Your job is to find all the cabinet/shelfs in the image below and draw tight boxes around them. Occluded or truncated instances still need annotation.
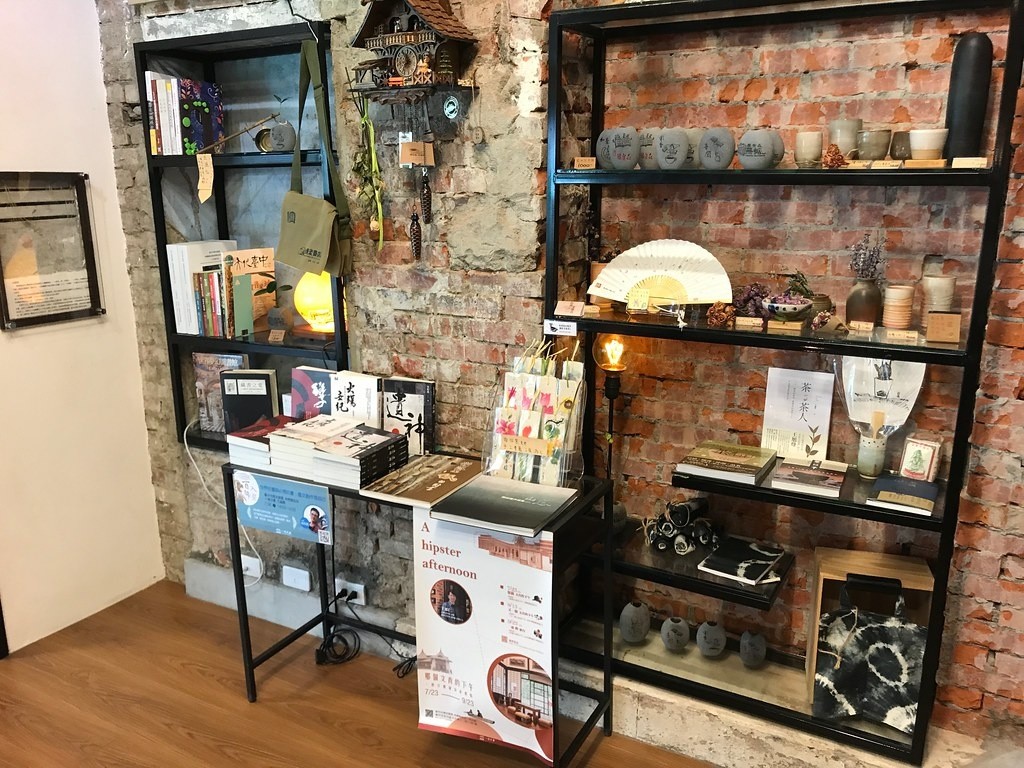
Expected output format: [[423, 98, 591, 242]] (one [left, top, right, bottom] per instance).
[[132, 18, 351, 459], [541, 1, 1023, 766], [220, 449, 615, 767]]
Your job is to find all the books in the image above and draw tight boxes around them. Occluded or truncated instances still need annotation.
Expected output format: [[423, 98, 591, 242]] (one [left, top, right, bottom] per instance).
[[166, 240, 278, 341], [696, 536, 785, 587], [737, 569, 781, 587], [144, 68, 225, 156], [191, 351, 581, 538], [865, 474, 939, 516], [760, 367, 836, 460], [678, 439, 777, 484], [771, 456, 850, 498]]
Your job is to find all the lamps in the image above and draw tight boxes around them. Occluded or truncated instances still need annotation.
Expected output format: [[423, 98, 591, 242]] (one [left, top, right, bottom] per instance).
[[590, 329, 636, 480], [293, 267, 335, 334]]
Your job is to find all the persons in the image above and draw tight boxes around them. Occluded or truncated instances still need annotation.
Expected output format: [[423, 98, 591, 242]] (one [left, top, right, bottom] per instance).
[[440, 589, 466, 624]]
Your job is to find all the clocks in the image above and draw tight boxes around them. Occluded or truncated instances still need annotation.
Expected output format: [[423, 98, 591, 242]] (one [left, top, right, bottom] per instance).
[[394, 45, 418, 78]]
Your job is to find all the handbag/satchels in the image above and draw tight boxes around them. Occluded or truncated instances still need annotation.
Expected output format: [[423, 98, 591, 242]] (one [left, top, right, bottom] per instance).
[[275, 191, 354, 278]]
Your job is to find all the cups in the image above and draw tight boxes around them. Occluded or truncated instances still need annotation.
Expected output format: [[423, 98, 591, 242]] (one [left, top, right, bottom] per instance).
[[910, 127, 950, 159], [920, 275, 958, 332], [856, 129, 892, 159], [795, 132, 823, 168], [882, 283, 915, 329], [858, 434, 888, 479], [828, 118, 863, 160]]
